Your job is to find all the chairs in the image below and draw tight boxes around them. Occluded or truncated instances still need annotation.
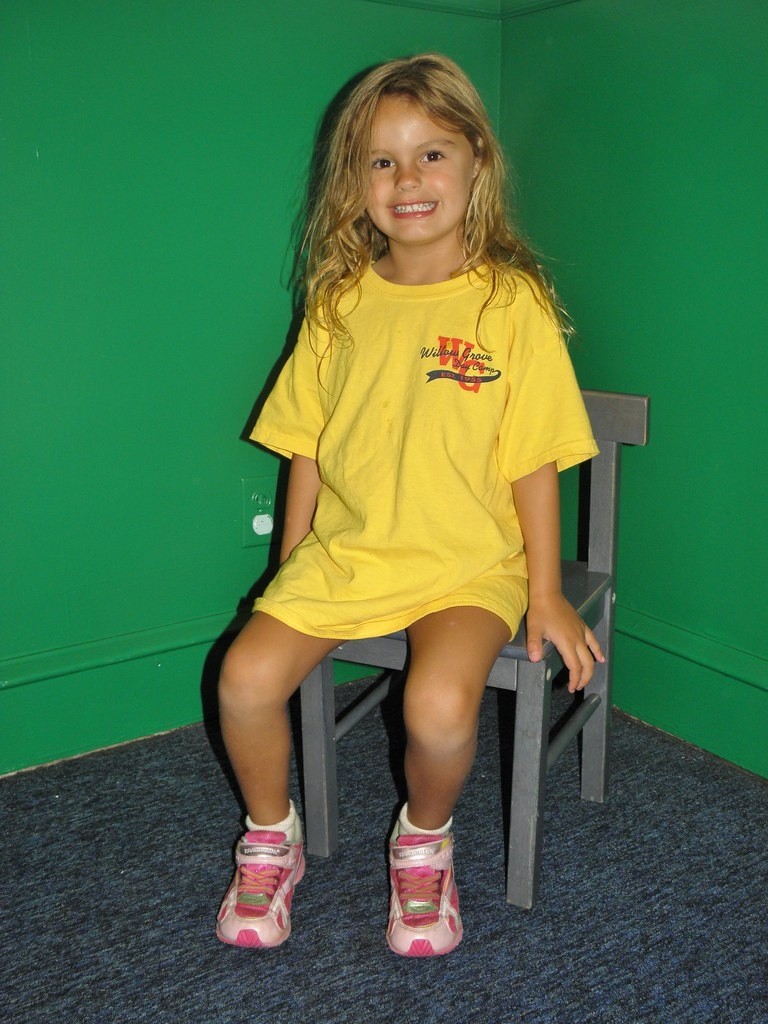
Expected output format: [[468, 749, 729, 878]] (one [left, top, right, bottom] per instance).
[[301, 389, 651, 910]]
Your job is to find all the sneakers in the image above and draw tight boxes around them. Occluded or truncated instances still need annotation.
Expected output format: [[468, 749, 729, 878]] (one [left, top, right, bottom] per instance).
[[385, 818, 463, 957], [215, 812, 306, 949]]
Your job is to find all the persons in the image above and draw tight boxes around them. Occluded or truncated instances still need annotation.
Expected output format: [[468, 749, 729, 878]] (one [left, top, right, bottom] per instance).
[[216, 53, 608, 958]]
